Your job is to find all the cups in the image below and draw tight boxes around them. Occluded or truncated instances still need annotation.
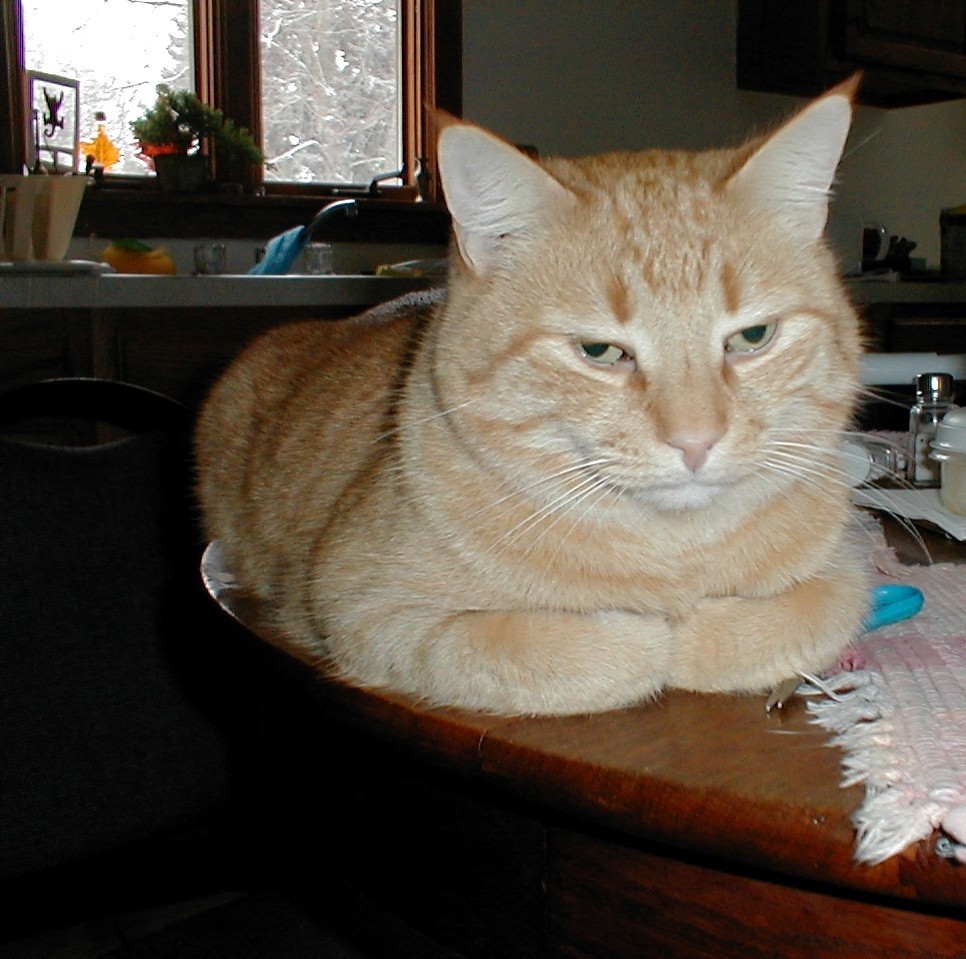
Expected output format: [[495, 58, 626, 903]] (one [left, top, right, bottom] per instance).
[[827, 200, 889, 276]]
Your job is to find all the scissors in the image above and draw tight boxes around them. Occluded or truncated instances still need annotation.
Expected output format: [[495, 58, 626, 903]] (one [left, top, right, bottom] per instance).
[[764, 582, 924, 710]]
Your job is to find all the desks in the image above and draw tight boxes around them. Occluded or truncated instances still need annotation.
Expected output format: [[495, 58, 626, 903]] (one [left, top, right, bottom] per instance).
[[201, 433, 965, 959]]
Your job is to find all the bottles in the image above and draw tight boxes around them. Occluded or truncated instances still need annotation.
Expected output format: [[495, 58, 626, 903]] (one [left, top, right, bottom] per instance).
[[843, 440, 904, 487], [907, 372, 959, 488]]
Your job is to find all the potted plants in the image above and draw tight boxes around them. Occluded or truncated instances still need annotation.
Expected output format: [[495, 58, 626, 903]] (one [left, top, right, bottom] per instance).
[[126, 87, 263, 192]]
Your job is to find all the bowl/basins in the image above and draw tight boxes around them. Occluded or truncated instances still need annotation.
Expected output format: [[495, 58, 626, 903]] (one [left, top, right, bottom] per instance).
[[928, 407, 966, 516]]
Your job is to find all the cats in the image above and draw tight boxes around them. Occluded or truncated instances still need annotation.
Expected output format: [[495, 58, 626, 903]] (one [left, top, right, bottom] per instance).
[[193, 70, 946, 719]]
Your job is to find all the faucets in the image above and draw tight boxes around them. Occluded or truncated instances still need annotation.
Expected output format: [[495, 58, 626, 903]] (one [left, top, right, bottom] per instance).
[[255, 197, 358, 273]]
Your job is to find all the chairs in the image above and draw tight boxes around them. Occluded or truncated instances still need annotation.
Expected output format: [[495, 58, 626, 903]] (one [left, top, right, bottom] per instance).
[[0, 377, 392, 959]]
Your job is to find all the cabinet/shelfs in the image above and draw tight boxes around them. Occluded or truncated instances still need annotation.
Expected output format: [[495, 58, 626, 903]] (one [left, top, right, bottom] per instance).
[[735, 0, 966, 109]]
